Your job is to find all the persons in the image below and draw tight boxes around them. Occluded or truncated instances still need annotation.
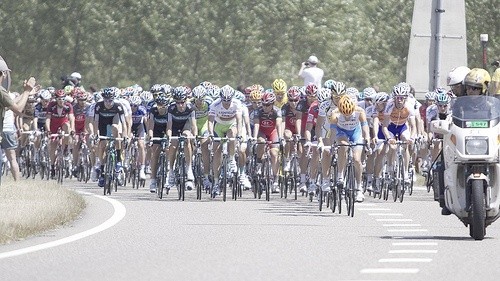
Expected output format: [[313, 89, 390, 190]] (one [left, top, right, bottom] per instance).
[[298, 55, 325, 85], [0, 56, 500, 201]]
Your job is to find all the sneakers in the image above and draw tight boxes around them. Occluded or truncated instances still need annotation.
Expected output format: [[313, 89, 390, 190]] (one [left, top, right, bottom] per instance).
[[0, 154, 453, 215]]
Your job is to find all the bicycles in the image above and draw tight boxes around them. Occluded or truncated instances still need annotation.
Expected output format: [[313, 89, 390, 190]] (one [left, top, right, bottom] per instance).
[[2, 126, 431, 218]]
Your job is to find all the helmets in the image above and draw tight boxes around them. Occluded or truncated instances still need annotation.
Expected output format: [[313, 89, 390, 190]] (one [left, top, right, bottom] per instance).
[[0, 55, 500, 117]]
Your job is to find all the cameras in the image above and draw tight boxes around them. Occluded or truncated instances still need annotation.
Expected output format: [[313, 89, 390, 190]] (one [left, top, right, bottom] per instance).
[[62, 76, 77, 86], [305, 62, 309, 65]]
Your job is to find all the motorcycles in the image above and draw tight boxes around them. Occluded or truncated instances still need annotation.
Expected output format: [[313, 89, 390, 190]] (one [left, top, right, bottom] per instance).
[[426, 95, 500, 240]]
